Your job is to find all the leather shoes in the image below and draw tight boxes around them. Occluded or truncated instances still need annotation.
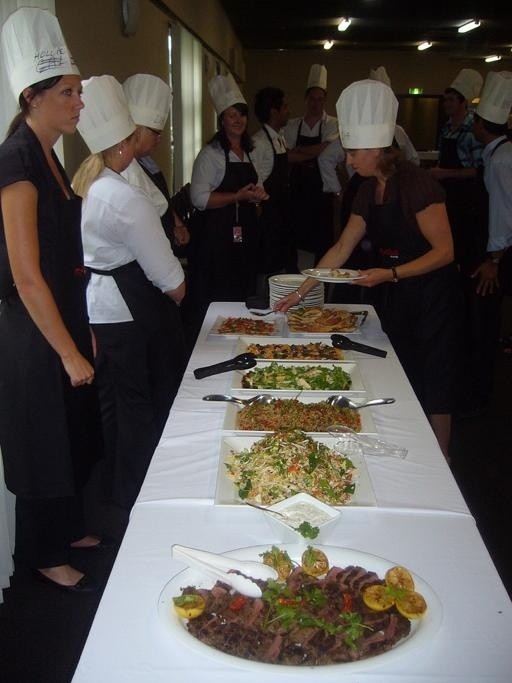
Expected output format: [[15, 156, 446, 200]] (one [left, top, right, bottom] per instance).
[[63, 536, 113, 553], [23, 568, 99, 597]]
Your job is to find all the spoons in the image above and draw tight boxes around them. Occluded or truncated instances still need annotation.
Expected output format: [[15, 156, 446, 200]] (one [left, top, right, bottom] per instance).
[[202, 394, 279, 407], [249, 311, 276, 316], [326, 395, 395, 410]]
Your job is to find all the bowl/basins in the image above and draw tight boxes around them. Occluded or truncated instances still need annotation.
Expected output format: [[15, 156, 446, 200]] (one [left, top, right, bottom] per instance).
[[263, 493, 342, 543]]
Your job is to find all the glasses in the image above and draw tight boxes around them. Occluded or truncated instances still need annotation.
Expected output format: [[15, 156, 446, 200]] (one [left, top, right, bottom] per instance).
[[145, 127, 161, 137]]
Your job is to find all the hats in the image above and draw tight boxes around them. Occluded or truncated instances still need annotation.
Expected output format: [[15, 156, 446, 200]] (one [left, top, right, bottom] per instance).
[[307, 63, 327, 90], [123, 72, 173, 130], [1, 6, 80, 108], [366, 65, 392, 89], [207, 71, 247, 119], [76, 74, 137, 154], [335, 78, 399, 149], [450, 68, 482, 102], [475, 70, 512, 124]]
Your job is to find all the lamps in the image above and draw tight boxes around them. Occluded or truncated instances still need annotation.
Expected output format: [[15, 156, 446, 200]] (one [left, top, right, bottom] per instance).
[[484, 54, 502, 64], [457, 18, 482, 34], [417, 40, 432, 51], [323, 39, 336, 50], [337, 14, 351, 32]]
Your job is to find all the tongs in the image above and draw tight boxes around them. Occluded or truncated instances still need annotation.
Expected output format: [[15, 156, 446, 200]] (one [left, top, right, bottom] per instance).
[[194, 352, 256, 380], [329, 333, 389, 359], [168, 542, 279, 601]]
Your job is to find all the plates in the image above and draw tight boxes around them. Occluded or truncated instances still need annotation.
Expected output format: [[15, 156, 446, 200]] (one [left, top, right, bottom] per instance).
[[232, 337, 356, 363], [269, 274, 326, 310], [222, 394, 379, 437], [230, 360, 367, 394], [212, 434, 382, 509], [208, 313, 286, 336], [301, 269, 368, 284], [157, 542, 442, 679], [285, 309, 362, 334]]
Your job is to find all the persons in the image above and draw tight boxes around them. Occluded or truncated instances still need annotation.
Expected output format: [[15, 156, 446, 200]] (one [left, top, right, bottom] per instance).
[[0, 5, 190, 595], [192, 65, 510, 466]]
